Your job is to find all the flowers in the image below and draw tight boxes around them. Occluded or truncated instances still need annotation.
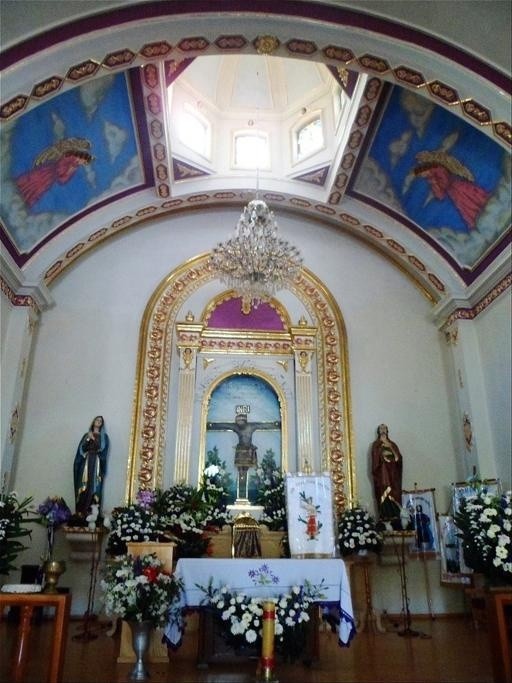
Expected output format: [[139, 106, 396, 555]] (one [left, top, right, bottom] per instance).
[[340, 504, 385, 555], [35, 494, 72, 589], [197, 579, 324, 652], [103, 553, 183, 625], [106, 465, 228, 560], [457, 486, 511, 584], [255, 467, 293, 557]]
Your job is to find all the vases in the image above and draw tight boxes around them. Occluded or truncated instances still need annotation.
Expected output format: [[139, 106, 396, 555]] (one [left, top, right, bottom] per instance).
[[127, 621, 157, 682], [40, 557, 67, 595], [125, 541, 178, 577]]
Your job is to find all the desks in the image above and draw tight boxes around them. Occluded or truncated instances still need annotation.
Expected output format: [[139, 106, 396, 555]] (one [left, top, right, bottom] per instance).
[[176, 556, 346, 670], [0, 588, 73, 683]]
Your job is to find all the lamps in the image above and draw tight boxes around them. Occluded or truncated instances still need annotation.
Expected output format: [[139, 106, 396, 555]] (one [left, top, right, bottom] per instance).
[[207, 168, 306, 310]]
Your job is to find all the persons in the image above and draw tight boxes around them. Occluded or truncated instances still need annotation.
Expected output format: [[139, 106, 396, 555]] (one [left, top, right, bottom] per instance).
[[205, 414, 280, 496], [73, 416, 110, 525], [370, 424, 403, 531], [407, 505, 434, 549]]
